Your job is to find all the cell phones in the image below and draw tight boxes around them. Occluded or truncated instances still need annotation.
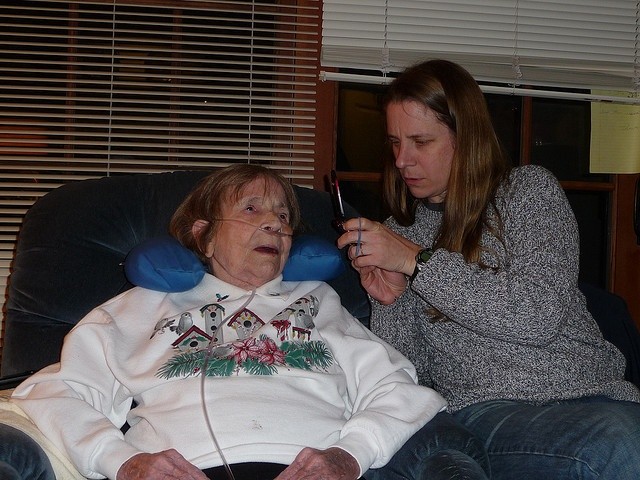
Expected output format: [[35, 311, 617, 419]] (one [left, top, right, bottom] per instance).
[[331, 170, 358, 250]]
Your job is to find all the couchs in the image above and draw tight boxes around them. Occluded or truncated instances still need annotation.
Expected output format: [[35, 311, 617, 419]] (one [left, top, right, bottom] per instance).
[[1, 167, 640, 480]]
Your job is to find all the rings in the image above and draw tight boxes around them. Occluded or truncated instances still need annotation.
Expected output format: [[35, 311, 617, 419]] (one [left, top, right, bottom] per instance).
[[360, 243, 365, 257]]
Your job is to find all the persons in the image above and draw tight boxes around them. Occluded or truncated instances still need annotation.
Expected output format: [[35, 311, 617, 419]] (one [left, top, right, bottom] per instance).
[[10, 163, 448, 479], [338, 60, 640, 480]]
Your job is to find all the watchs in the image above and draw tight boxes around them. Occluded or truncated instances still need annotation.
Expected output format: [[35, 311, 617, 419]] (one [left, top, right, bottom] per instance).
[[410, 246, 434, 283]]
